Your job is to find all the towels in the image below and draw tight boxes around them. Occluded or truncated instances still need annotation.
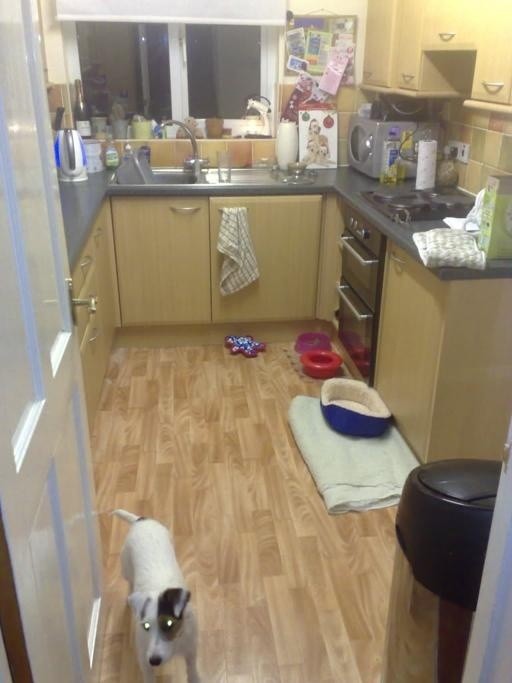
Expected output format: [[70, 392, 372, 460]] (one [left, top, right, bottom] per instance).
[[382, 460, 504, 683]]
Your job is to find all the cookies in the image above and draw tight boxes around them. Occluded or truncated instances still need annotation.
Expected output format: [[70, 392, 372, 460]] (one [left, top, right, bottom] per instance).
[[346, 114, 441, 180]]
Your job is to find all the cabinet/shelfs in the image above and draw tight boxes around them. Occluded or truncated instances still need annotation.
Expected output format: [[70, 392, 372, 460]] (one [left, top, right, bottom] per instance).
[[106, 140, 151, 169], [463, 0, 512, 108], [72, 79, 92, 139], [357, 3, 467, 101], [380, 128, 400, 187], [373, 232, 512, 468], [106, 166, 334, 345], [421, 1, 479, 50], [57, 183, 116, 417]]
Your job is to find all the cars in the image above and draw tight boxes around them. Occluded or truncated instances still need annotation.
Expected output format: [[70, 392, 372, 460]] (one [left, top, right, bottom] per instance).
[[91, 115, 164, 140], [274, 122, 299, 172], [205, 117, 224, 139], [217, 150, 233, 181], [288, 162, 307, 182]]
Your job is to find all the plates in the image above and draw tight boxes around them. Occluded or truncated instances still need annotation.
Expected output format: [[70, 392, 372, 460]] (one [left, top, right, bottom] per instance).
[[373, 232, 512, 468], [463, 0, 512, 108], [357, 3, 467, 101], [421, 1, 479, 50]]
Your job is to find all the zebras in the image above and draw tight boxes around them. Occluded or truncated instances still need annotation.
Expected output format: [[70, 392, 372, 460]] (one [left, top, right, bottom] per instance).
[[335, 231, 384, 387]]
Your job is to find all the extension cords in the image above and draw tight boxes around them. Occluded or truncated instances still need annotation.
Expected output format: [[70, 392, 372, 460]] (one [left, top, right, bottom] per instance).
[[54, 129, 89, 183]]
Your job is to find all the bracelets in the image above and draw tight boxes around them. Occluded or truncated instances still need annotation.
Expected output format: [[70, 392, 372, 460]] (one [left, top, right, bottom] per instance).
[[339, 329, 371, 377], [296, 331, 343, 379]]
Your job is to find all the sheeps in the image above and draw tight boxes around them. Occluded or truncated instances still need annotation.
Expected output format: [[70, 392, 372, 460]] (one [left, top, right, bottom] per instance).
[[153, 119, 199, 159]]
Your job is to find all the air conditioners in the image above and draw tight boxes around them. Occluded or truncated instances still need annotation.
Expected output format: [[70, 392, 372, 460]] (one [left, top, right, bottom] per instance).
[[108, 509, 200, 683]]
